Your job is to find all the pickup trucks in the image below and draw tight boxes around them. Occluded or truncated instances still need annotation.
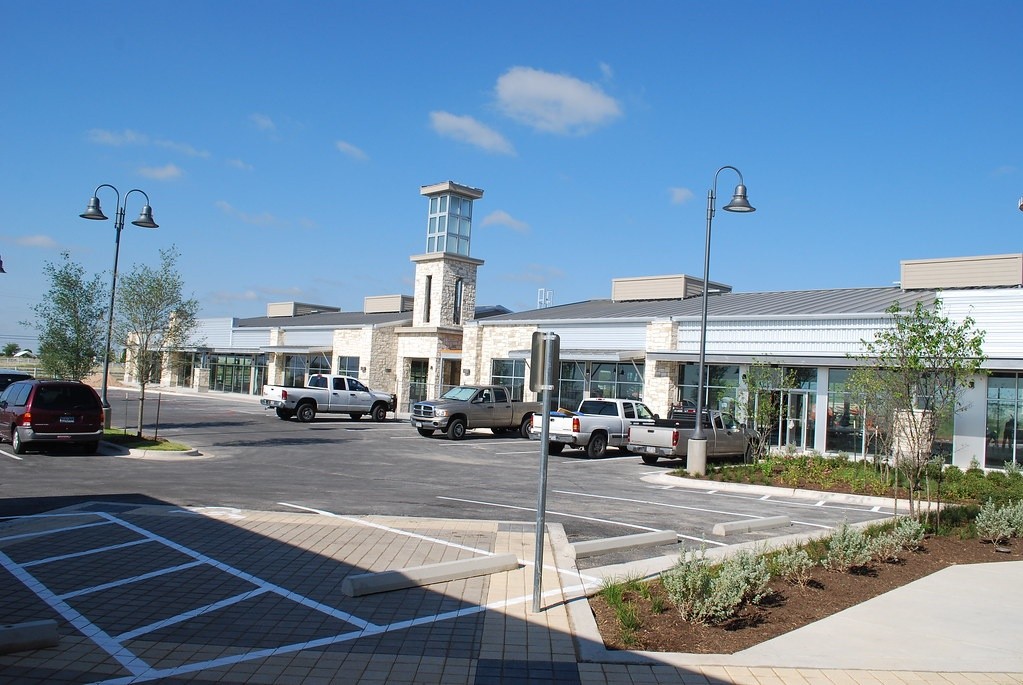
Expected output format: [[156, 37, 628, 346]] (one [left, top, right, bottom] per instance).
[[527, 396, 662, 459], [625, 402, 761, 467], [408, 384, 545, 441], [259, 373, 399, 424]]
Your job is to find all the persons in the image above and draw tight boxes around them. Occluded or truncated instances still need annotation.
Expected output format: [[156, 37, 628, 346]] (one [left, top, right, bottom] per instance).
[[1002, 418, 1014, 448]]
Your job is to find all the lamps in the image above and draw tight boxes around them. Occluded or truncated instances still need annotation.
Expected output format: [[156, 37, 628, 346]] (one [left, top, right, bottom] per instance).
[[361, 367, 366, 370], [463, 369, 470, 373]]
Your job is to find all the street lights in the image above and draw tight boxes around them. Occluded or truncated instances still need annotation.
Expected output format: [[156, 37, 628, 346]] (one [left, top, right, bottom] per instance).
[[77, 183, 159, 408], [691, 165, 757, 440]]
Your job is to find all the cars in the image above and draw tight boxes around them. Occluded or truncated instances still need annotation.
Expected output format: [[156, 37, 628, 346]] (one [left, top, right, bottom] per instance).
[[0, 367, 34, 397]]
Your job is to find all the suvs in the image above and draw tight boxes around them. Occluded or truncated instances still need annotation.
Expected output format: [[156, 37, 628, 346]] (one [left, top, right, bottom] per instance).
[[0, 377, 105, 454]]
